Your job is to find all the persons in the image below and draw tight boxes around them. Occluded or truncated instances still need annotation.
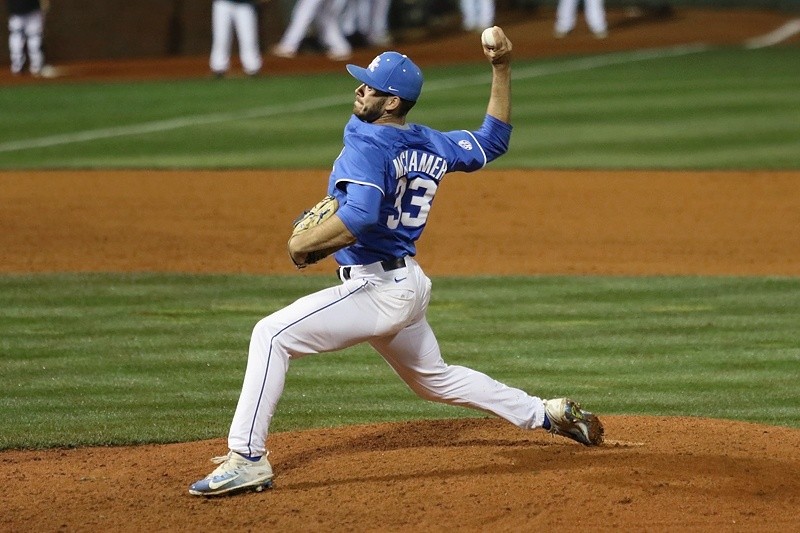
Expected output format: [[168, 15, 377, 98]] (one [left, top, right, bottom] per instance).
[[6, 0, 49, 75], [210, 0, 395, 78], [552, 0, 609, 41], [189, 26, 605, 497], [459, 0, 496, 34]]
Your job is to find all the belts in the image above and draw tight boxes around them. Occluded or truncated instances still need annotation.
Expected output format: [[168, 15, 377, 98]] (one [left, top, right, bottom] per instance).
[[337, 258, 407, 279]]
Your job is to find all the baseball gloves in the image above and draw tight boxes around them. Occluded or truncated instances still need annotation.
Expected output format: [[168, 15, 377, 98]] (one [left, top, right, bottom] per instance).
[[288, 194, 355, 266]]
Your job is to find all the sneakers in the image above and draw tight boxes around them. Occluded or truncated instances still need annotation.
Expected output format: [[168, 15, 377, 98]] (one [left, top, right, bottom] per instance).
[[543, 398, 605, 448], [189, 452, 275, 499]]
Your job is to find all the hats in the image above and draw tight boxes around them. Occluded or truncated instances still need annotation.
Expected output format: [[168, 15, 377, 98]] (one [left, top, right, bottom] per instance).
[[346, 51, 423, 102]]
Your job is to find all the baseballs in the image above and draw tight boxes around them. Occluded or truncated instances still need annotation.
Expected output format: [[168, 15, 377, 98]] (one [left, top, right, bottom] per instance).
[[481, 27, 501, 50]]
